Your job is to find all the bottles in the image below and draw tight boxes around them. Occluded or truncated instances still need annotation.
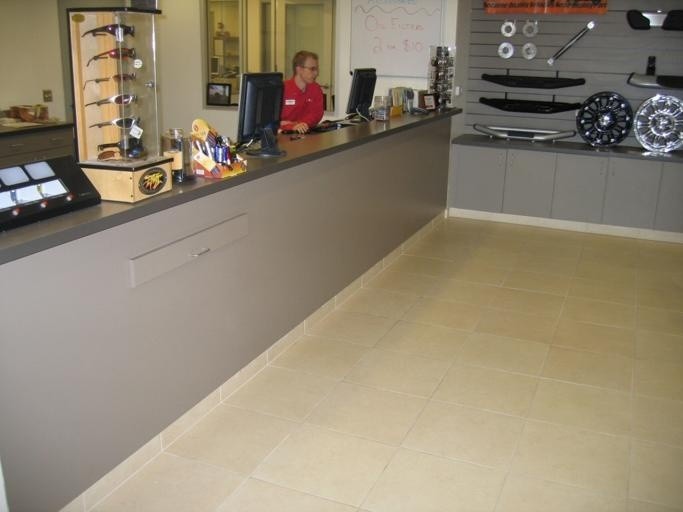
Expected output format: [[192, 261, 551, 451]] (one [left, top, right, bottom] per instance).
[[374, 96, 390, 121], [166, 127, 185, 184]]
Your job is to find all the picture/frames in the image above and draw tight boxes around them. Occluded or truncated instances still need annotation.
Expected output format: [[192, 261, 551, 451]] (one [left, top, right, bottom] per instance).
[[207, 83, 232, 106]]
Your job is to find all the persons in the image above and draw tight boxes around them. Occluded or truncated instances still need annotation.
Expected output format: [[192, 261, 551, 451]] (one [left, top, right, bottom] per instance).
[[277, 48, 327, 136]]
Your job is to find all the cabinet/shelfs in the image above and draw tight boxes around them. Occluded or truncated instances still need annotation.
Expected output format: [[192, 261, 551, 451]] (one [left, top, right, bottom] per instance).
[[212, 37, 239, 75], [0, 123, 74, 169]]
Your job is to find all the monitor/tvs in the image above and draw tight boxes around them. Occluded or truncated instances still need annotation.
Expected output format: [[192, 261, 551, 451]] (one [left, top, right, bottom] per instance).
[[236, 72, 287, 158], [346, 68, 377, 123], [211, 56, 220, 75]]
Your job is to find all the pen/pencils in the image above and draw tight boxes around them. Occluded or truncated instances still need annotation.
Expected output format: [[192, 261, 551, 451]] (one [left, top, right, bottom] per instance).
[[195, 140, 231, 167]]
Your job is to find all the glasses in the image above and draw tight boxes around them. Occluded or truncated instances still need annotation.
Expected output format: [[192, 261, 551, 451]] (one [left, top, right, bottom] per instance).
[[86, 48, 137, 67], [298, 65, 319, 71], [98, 138, 145, 160], [82, 24, 135, 38], [89, 117, 144, 130], [85, 94, 137, 107], [83, 71, 136, 90]]
